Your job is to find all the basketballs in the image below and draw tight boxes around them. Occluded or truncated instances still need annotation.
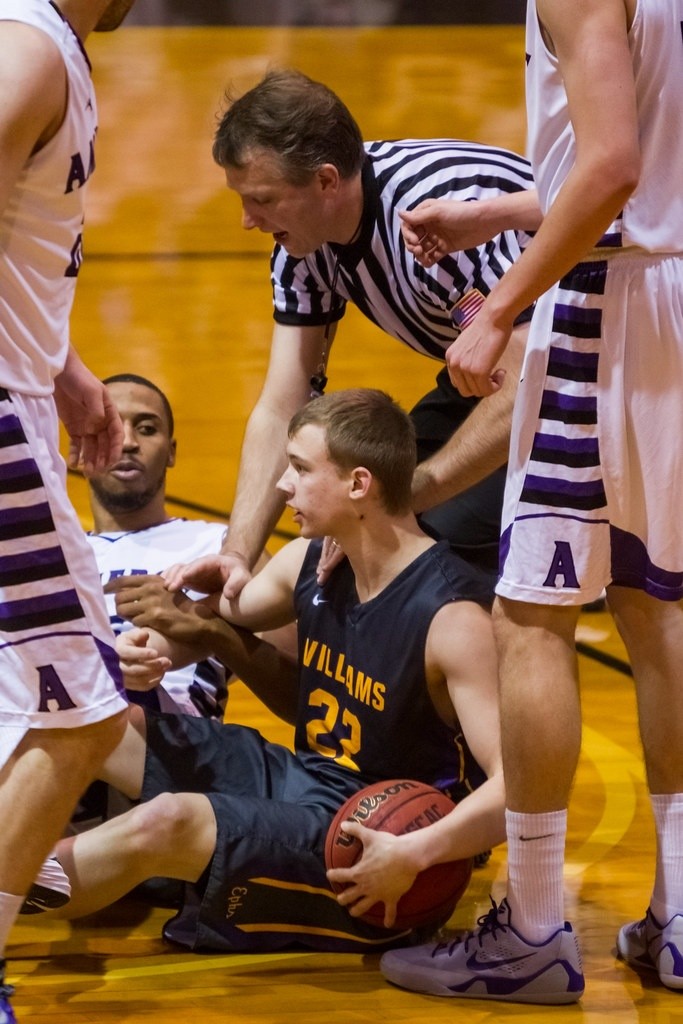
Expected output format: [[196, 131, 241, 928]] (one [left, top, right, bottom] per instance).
[[325, 777, 473, 932]]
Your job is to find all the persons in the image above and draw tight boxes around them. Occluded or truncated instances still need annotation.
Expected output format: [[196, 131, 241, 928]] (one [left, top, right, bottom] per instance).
[[0, 0, 683, 1024]]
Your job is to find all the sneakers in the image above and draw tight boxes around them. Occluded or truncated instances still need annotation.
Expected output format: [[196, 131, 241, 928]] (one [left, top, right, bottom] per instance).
[[380, 894, 585, 1005], [19, 852, 71, 915], [0, 959, 17, 1024], [616, 907, 683, 989]]
